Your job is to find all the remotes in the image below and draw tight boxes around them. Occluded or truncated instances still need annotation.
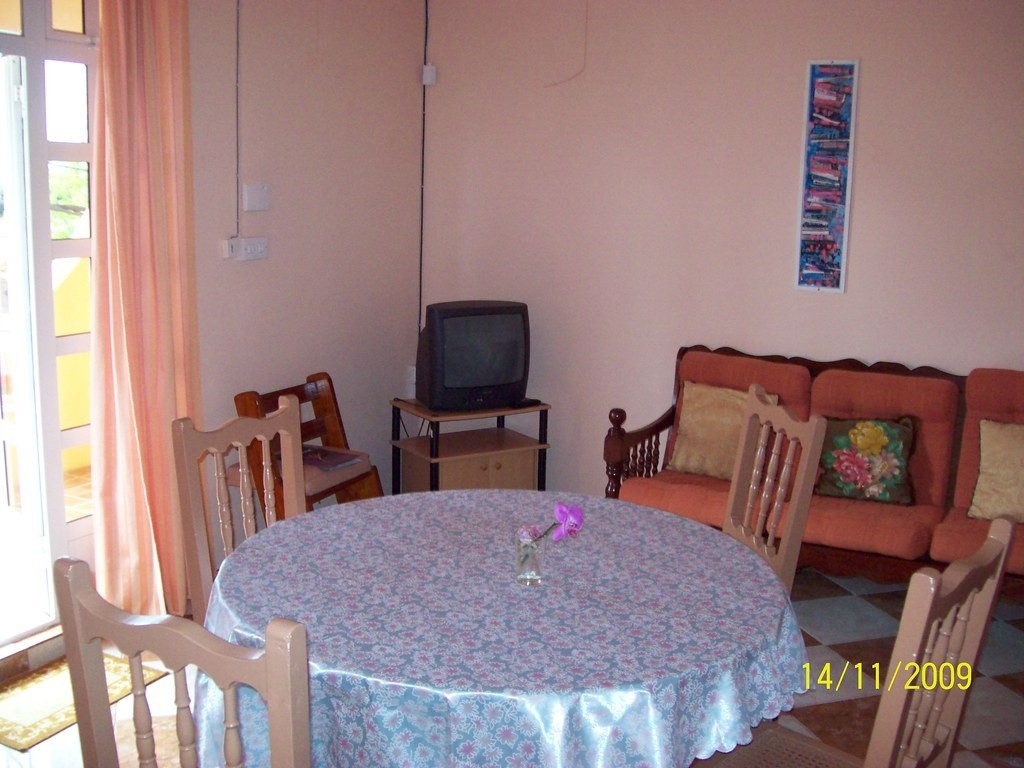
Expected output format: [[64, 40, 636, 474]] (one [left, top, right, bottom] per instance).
[[512, 398, 542, 410]]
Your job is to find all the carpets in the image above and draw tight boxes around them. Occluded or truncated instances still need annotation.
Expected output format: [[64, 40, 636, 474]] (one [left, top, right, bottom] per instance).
[[0, 652, 169, 752]]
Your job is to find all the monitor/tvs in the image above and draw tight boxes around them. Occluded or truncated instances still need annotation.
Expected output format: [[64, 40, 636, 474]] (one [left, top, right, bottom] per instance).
[[416, 300, 529, 411]]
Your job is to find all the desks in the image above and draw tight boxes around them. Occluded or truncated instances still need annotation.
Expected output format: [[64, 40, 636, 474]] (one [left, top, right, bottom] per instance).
[[193, 489, 816, 768]]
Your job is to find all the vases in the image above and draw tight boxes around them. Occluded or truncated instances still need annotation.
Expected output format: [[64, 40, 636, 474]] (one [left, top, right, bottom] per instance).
[[517, 538, 545, 586]]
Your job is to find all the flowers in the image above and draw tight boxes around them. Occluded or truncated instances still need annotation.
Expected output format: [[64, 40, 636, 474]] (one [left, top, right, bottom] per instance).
[[518, 504, 584, 566]]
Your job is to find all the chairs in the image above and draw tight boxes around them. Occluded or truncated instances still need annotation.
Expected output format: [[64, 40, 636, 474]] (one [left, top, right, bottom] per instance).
[[172, 394, 306, 626], [689, 515, 1018, 768], [723, 383, 828, 594], [54, 557, 310, 768], [227, 372, 384, 529]]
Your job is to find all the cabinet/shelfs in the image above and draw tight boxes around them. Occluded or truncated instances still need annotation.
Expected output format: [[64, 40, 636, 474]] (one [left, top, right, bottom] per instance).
[[389, 398, 552, 495]]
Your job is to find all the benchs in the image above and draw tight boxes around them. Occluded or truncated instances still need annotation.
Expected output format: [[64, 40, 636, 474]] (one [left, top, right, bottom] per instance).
[[604, 345, 1023, 601]]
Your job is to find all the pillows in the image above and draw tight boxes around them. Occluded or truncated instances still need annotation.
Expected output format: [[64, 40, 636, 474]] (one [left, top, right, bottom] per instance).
[[665, 381, 778, 481], [814, 417, 914, 505], [967, 419, 1024, 524]]
